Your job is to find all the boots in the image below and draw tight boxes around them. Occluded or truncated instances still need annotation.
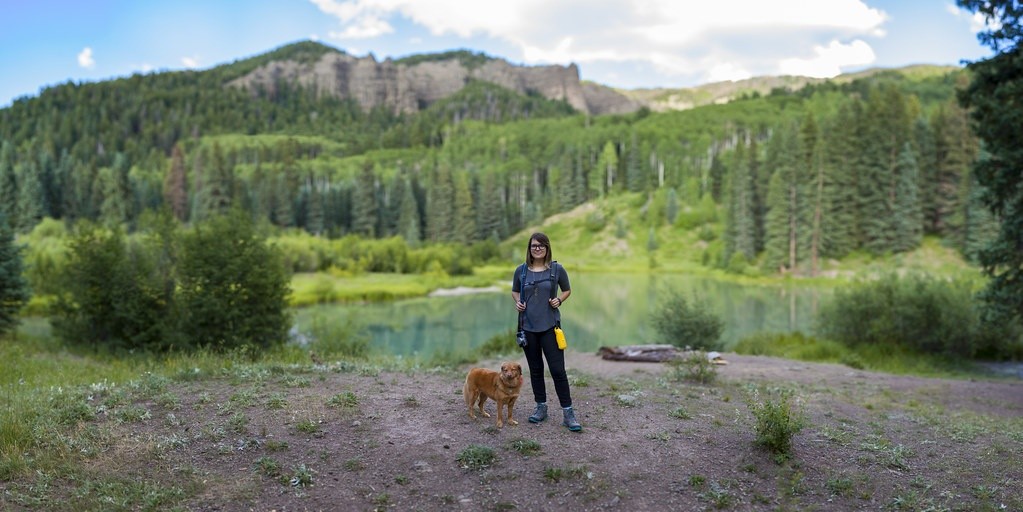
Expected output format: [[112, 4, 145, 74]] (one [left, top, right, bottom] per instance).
[[528, 403, 548, 423], [562, 407, 581, 431]]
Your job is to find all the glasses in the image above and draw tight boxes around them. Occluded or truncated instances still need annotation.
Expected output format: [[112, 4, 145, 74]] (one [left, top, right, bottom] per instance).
[[530, 244, 547, 250]]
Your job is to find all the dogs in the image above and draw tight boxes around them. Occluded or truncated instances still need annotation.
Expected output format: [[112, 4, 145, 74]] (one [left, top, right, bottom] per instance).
[[462, 361, 523, 428]]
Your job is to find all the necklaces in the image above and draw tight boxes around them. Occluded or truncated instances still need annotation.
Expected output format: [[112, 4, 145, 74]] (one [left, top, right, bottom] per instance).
[[532, 264, 545, 296]]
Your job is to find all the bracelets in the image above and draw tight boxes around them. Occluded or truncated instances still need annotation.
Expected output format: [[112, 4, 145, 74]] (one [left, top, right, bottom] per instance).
[[556, 297, 562, 307]]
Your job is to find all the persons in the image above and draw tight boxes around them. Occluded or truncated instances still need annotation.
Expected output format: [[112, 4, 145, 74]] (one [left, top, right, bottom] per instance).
[[512, 232, 583, 431]]
